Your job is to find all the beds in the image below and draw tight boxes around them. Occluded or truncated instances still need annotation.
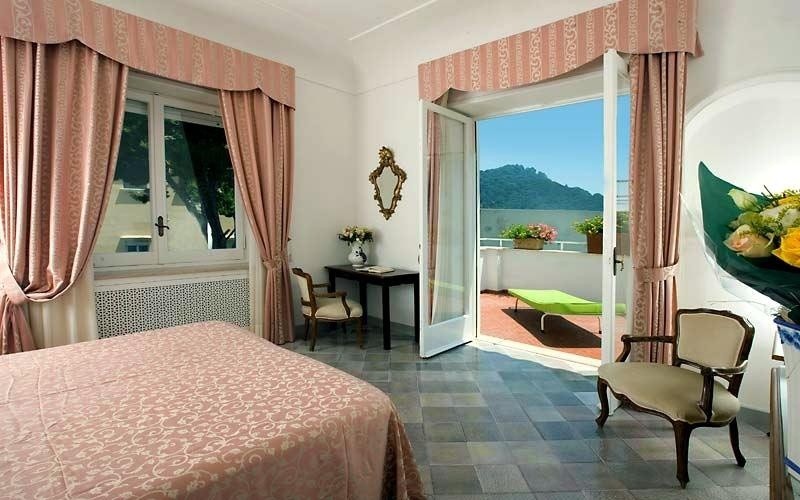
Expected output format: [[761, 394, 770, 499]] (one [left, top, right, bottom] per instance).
[[0, 318, 430, 500]]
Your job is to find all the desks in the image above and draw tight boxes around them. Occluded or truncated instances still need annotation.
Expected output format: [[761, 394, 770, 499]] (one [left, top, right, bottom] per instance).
[[323, 263, 420, 350]]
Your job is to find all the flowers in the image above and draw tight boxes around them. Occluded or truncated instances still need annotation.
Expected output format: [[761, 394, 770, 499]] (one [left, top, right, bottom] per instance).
[[335, 223, 375, 248], [497, 221, 560, 244], [724, 182, 800, 273], [571, 210, 629, 237]]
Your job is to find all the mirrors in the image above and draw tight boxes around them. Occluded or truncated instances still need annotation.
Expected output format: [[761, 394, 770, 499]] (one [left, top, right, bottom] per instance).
[[368, 145, 408, 222]]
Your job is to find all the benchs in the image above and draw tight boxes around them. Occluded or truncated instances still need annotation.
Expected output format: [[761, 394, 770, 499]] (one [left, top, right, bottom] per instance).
[[507, 288, 627, 334]]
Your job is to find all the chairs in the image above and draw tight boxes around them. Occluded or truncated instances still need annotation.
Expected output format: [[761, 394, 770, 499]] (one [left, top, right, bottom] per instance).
[[291, 267, 363, 352], [595, 305, 756, 489]]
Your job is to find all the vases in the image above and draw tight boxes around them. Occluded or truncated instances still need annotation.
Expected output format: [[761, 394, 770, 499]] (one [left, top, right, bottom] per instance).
[[347, 240, 368, 269], [587, 233, 630, 256], [770, 312, 800, 483], [513, 235, 544, 250]]
